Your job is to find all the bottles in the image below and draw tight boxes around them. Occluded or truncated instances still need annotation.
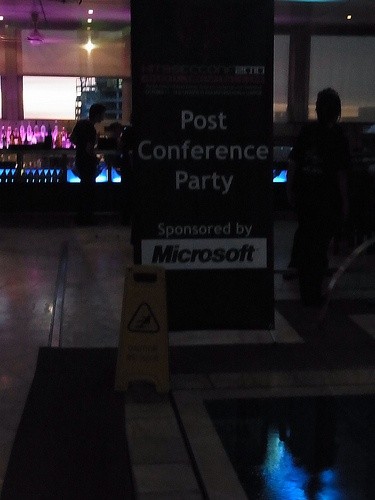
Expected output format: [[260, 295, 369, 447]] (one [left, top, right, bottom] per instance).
[[0, 169, 58, 184], [0, 120, 68, 150]]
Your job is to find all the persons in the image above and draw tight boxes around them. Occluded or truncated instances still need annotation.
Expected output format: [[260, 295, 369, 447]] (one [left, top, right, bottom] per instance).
[[274, 85, 364, 315], [68, 103, 109, 229], [110, 112, 135, 224]]
